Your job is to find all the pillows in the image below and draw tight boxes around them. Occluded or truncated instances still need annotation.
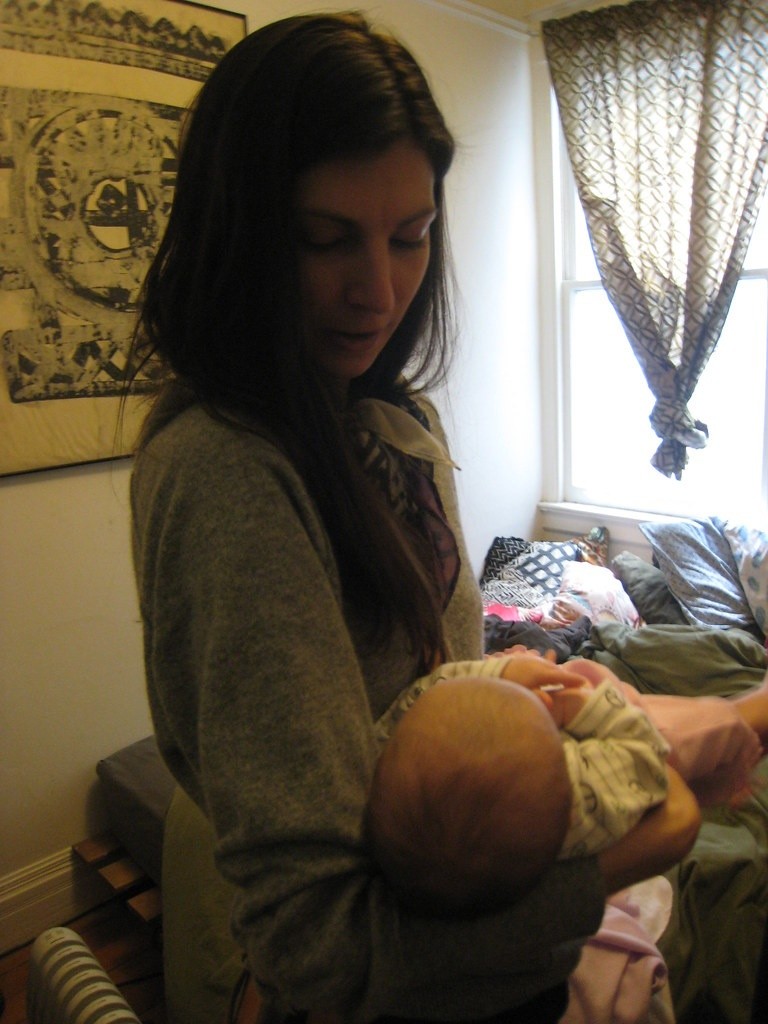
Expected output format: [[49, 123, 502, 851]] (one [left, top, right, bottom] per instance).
[[479, 523, 610, 614]]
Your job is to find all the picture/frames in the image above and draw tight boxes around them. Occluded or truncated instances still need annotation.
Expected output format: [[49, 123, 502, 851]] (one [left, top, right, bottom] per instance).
[[0, 1, 248, 480]]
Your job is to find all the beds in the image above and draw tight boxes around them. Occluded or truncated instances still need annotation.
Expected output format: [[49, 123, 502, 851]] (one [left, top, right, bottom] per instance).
[[68, 509, 768, 1024]]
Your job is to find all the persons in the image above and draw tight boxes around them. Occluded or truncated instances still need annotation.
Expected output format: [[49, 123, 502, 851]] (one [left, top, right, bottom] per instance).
[[107, 9, 768, 1024]]
